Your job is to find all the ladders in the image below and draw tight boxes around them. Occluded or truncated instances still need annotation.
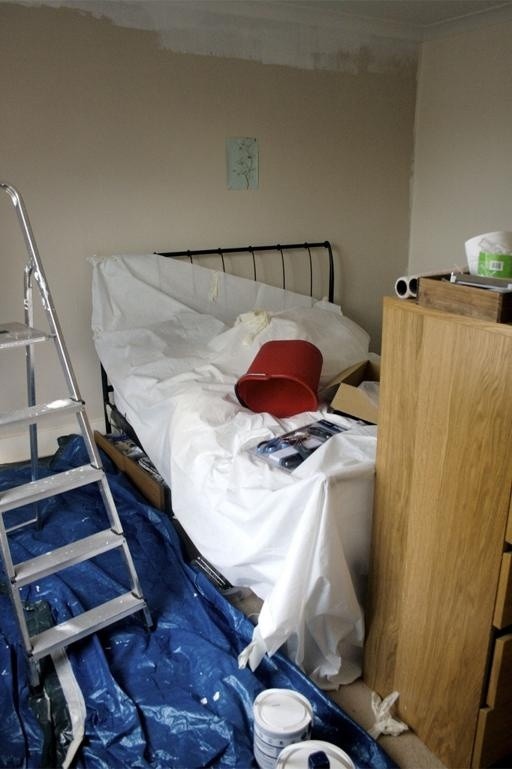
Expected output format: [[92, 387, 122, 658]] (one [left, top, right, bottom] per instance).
[[0, 183, 154, 698]]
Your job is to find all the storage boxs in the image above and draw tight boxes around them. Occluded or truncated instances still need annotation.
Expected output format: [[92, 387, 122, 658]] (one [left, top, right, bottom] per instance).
[[415, 271, 512, 324], [317, 357, 380, 426]]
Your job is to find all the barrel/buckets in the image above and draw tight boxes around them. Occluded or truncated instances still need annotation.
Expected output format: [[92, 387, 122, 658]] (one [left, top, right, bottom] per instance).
[[236, 340, 325, 418]]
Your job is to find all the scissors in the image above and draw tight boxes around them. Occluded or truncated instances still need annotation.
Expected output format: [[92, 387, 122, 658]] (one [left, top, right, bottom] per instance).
[[256, 437, 307, 453]]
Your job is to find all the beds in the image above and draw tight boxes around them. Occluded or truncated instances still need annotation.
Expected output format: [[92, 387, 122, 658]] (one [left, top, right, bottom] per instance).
[[89, 240, 379, 691]]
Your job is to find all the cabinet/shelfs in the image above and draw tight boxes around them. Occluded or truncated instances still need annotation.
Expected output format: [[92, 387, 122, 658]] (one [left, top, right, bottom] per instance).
[[358, 293, 512, 769]]
